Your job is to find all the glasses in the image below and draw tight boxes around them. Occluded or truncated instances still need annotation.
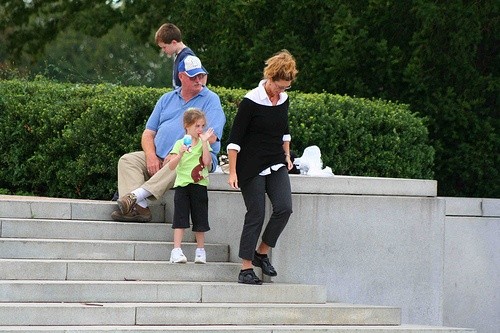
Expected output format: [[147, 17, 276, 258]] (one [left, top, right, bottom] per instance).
[[273, 80, 291, 89], [190, 74, 204, 81]]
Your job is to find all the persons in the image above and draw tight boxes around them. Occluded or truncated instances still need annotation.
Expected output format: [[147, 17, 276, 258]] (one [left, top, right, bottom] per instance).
[[224, 51, 297, 285], [110, 55, 227, 222], [155, 23, 210, 91], [168, 108, 214, 264]]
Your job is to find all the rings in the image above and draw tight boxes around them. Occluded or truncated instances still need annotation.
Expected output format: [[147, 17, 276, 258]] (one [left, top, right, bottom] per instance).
[[147, 169, 149, 172]]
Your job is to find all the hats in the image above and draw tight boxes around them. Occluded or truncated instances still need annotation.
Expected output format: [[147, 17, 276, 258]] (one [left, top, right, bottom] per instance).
[[178, 54, 206, 77]]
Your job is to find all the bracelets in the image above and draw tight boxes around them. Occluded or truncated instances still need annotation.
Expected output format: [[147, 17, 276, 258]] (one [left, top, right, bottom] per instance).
[[284, 152, 291, 158]]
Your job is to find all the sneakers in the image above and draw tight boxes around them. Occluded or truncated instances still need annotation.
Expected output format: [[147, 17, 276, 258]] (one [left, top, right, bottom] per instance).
[[169, 248, 187, 263], [195, 247, 207, 264], [111, 203, 152, 222], [117, 192, 137, 214], [238, 268, 263, 285], [251, 250, 277, 276]]
